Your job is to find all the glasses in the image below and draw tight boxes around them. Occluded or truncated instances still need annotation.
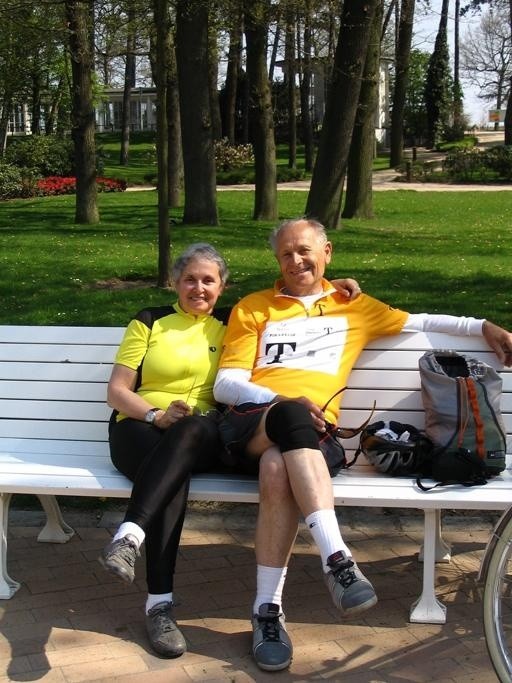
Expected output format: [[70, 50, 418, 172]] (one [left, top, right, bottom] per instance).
[[185, 375, 241, 423], [322, 385, 378, 440]]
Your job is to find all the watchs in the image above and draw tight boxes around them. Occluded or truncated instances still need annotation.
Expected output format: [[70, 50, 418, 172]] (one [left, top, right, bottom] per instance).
[[144, 408, 162, 425]]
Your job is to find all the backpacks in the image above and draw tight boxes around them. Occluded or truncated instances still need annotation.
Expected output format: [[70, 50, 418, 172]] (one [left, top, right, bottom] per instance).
[[415, 348, 508, 492]]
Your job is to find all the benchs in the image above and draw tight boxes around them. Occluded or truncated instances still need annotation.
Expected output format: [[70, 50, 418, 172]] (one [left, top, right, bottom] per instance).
[[0, 321, 512, 629]]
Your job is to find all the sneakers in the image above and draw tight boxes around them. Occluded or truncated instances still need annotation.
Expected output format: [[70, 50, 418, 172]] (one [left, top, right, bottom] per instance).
[[319, 550, 380, 615], [96, 538, 139, 585], [250, 601, 293, 672], [144, 599, 188, 658]]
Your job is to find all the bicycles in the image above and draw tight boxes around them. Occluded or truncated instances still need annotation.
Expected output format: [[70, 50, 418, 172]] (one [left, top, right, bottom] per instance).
[[476, 500, 512, 683]]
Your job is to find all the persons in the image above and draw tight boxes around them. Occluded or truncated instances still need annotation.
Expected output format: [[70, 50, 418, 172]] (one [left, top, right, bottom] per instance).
[[95, 242, 363, 660], [212, 218, 512, 672]]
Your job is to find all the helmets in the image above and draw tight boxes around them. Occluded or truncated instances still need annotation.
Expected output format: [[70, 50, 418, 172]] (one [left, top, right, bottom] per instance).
[[358, 420, 422, 477]]
